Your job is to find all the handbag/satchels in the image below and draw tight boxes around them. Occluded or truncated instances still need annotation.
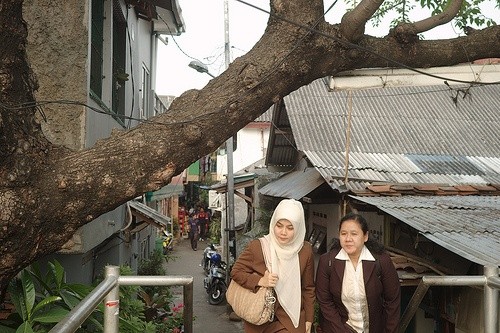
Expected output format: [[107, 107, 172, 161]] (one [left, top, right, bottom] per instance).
[[226, 238, 276, 325]]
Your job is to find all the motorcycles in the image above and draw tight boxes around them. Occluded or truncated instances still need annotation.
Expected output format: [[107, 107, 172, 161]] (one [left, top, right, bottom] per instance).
[[201, 243, 233, 305]]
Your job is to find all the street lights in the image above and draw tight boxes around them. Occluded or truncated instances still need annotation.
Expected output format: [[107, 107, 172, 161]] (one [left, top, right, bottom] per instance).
[[188, 61, 235, 284]]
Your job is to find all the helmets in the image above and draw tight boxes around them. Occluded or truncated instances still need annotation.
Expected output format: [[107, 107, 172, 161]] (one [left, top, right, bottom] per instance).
[[211, 253, 221, 266]]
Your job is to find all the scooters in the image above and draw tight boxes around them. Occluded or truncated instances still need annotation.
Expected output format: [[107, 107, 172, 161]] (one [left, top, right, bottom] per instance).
[[163, 230, 173, 255]]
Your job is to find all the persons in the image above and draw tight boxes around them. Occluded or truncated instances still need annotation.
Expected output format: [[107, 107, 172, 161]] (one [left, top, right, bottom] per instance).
[[184, 205, 209, 242], [315, 214, 402, 333], [188, 214, 201, 251], [230, 199, 315, 333]]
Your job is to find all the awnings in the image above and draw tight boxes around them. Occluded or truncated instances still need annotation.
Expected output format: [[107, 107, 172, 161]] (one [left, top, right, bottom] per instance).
[[258, 169, 325, 204], [200, 176, 254, 203], [130, 200, 171, 230]]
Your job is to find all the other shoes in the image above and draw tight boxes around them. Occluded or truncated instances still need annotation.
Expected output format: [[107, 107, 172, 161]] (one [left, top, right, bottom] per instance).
[[199, 238, 204, 241]]
